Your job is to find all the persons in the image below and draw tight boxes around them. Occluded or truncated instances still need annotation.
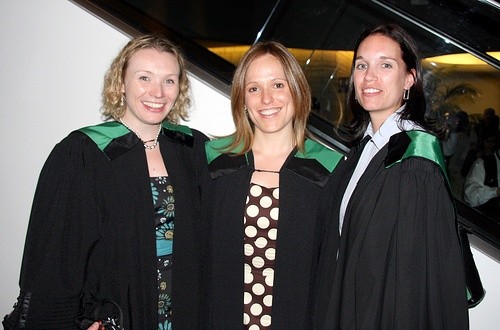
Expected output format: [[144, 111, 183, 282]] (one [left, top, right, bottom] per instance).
[[198, 41, 347, 329], [310, 23, 486, 330], [448, 109, 471, 181], [474, 107, 500, 146], [464, 134, 500, 220], [1, 34, 211, 330]]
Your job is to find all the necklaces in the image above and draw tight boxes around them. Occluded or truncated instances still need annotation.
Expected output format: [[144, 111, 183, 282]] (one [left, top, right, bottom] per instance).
[[119, 117, 163, 150]]
